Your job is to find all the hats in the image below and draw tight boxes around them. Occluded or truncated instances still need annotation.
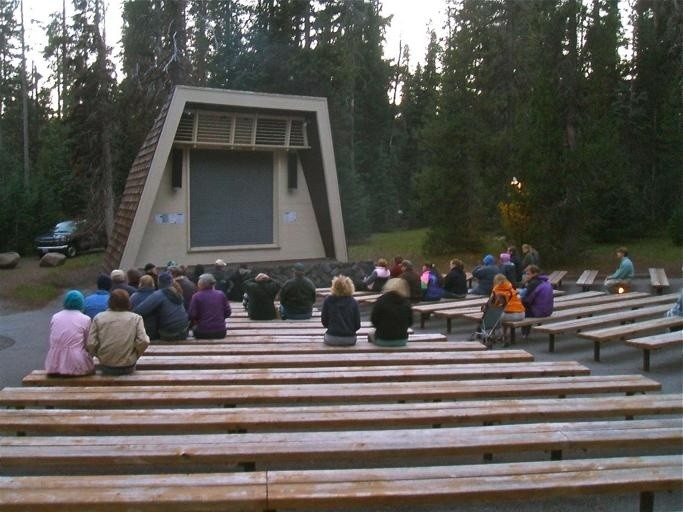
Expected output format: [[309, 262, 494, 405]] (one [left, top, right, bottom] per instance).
[[64, 259, 226, 310], [483, 256, 492, 264], [399, 260, 411, 266], [293, 263, 304, 270], [617, 247, 627, 252]]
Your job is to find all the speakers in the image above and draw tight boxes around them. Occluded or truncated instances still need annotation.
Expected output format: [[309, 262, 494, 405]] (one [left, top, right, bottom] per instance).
[[288, 153, 297, 189], [171, 147, 182, 187]]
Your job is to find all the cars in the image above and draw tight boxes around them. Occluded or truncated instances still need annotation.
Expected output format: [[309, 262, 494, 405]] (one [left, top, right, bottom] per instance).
[[36, 218, 106, 255]]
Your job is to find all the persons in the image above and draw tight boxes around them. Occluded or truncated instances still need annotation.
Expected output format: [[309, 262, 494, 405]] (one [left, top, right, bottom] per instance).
[[44, 289, 96, 377], [355, 244, 556, 339], [320, 275, 361, 347], [366, 278, 413, 348], [603, 246, 634, 295], [85, 288, 151, 376], [84, 258, 318, 340]]
